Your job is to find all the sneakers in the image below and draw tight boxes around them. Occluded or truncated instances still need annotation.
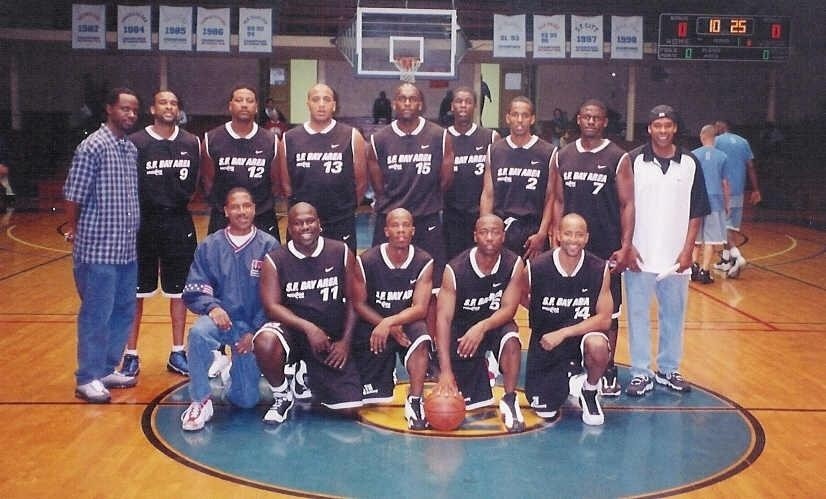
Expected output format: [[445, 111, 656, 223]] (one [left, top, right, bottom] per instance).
[[691, 257, 746, 283], [659, 372, 692, 391], [262, 391, 293, 425], [404, 394, 427, 429], [578, 383, 606, 424], [76, 349, 139, 404], [168, 349, 226, 376], [179, 398, 213, 431], [604, 368, 620, 394], [291, 362, 307, 396], [627, 373, 655, 396], [487, 347, 500, 386], [498, 397, 524, 430]]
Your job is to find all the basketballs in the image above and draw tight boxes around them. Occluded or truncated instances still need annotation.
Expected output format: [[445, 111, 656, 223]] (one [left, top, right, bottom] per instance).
[[423, 388, 466, 430]]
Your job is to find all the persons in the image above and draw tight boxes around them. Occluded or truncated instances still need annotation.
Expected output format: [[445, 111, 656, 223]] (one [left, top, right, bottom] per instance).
[[623, 103, 710, 398], [711, 119, 761, 280], [550, 98, 635, 399], [119, 90, 201, 378], [60, 84, 138, 405], [688, 124, 730, 284], [0, 73, 825, 231], [124, 184, 559, 435], [518, 212, 614, 427]]
